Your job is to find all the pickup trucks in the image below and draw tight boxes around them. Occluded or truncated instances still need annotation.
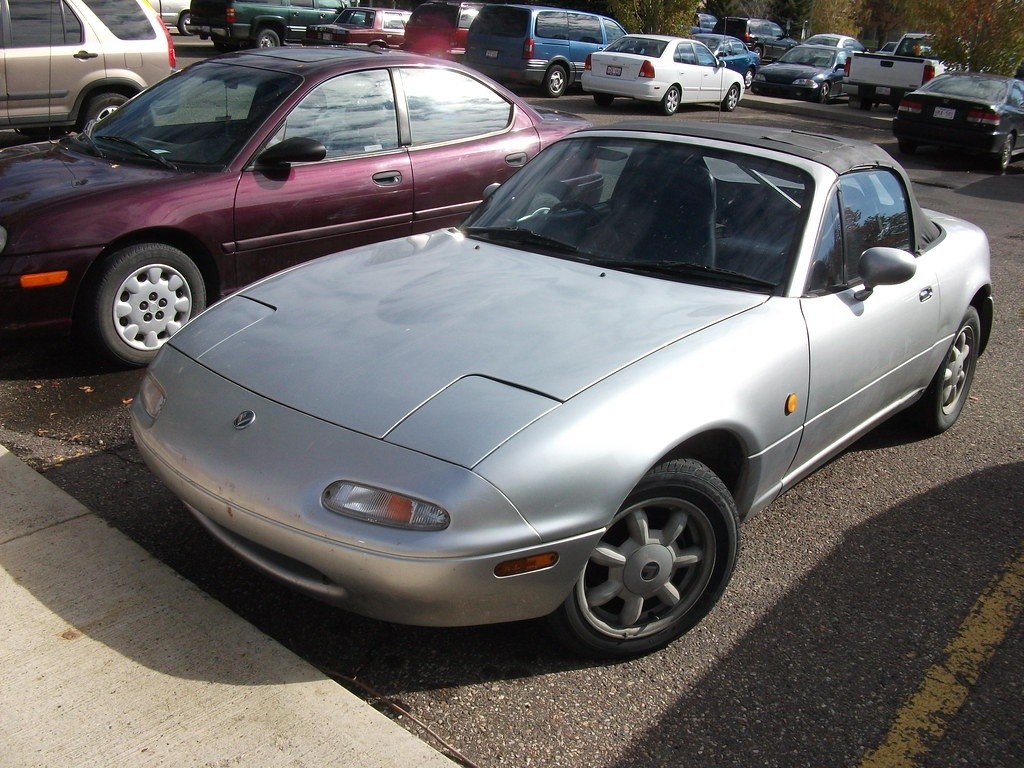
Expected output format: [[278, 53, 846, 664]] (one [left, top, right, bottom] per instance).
[[841, 31, 982, 110]]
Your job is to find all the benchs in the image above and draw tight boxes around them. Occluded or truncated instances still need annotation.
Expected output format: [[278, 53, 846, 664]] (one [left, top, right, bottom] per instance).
[[718, 180, 807, 283]]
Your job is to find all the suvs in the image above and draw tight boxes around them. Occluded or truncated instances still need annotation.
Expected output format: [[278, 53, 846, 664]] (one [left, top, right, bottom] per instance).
[[398, 0, 482, 54], [711, 17, 799, 66], [301, 6, 411, 48], [189, 0, 356, 54]]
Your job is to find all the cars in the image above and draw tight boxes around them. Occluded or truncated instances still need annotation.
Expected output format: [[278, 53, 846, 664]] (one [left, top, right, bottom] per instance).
[[685, 34, 761, 89], [874, 41, 934, 55], [748, 45, 853, 104], [1, 45, 604, 367], [147, 1, 196, 36], [799, 34, 870, 56], [580, 34, 746, 117], [891, 70, 1024, 171], [136, 118, 991, 661], [0, 0, 182, 136], [672, 13, 720, 37]]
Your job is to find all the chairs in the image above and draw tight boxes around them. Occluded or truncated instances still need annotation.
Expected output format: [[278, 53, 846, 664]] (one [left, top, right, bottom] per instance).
[[585, 142, 717, 269], [643, 46, 660, 58]]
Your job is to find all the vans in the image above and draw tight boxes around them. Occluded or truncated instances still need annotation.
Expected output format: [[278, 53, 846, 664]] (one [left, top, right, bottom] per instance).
[[463, 4, 645, 98]]
